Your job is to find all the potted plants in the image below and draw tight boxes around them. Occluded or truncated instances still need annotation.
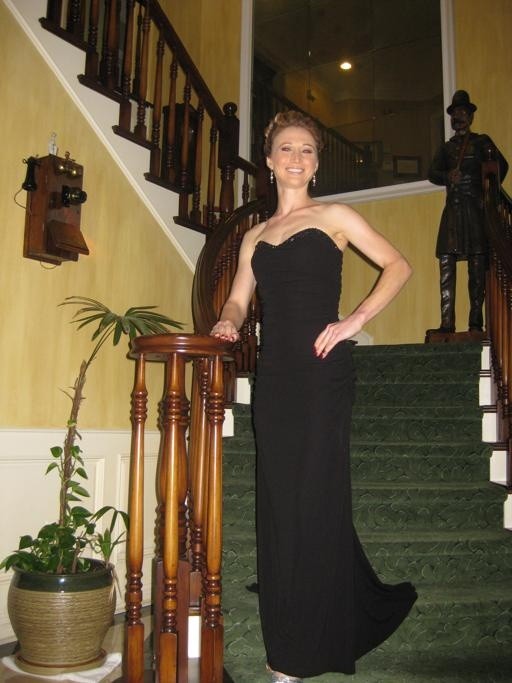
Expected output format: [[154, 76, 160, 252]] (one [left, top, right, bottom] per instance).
[[0, 295, 186, 676]]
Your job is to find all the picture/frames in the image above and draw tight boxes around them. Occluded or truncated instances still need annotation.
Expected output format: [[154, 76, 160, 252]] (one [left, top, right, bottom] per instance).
[[392, 154, 422, 178], [348, 141, 383, 168]]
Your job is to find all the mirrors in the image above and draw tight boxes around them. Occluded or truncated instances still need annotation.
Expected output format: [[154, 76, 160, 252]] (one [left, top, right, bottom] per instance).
[[237, 0, 456, 220]]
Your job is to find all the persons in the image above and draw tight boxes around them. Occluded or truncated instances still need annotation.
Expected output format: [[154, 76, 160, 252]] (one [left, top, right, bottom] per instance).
[[426, 91, 508, 335], [209, 110, 418, 683]]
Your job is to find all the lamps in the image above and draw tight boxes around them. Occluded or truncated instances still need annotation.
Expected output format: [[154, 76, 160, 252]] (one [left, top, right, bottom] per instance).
[[307, 37, 316, 103]]
